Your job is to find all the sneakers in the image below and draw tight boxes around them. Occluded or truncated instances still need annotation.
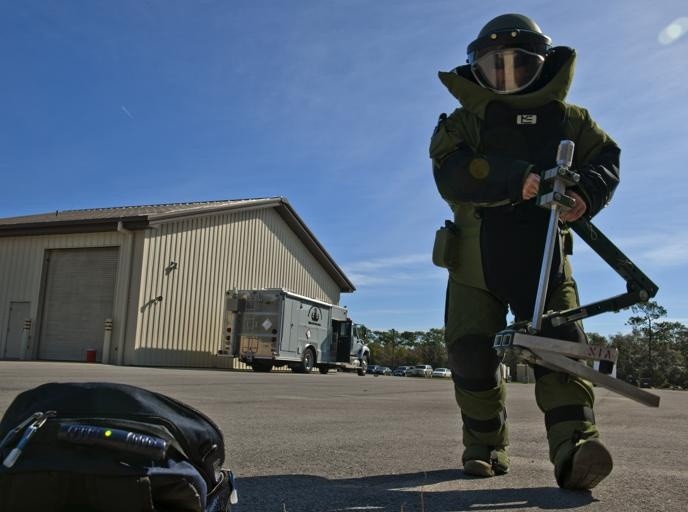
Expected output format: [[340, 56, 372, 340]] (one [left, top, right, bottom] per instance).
[[556, 440, 616, 495], [460, 458, 509, 477]]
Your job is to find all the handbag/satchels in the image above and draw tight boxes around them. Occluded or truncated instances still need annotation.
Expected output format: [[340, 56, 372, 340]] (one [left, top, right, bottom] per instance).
[[0, 377, 241, 512]]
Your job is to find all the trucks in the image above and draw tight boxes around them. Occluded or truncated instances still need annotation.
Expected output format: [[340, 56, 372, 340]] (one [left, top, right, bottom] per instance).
[[216, 287, 374, 375]]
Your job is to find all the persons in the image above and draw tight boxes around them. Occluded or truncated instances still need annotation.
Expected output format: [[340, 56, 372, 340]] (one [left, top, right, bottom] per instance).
[[429, 12, 622, 492]]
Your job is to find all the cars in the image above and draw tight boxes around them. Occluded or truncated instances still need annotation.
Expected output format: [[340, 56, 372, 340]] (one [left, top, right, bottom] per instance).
[[625, 372, 652, 388], [365, 364, 453, 378]]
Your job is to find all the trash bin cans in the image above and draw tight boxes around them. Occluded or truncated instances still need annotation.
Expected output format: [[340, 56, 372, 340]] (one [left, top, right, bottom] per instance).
[[86, 349, 97, 363]]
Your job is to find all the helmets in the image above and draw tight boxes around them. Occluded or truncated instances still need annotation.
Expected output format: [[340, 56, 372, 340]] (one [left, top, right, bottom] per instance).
[[463, 11, 554, 95]]
